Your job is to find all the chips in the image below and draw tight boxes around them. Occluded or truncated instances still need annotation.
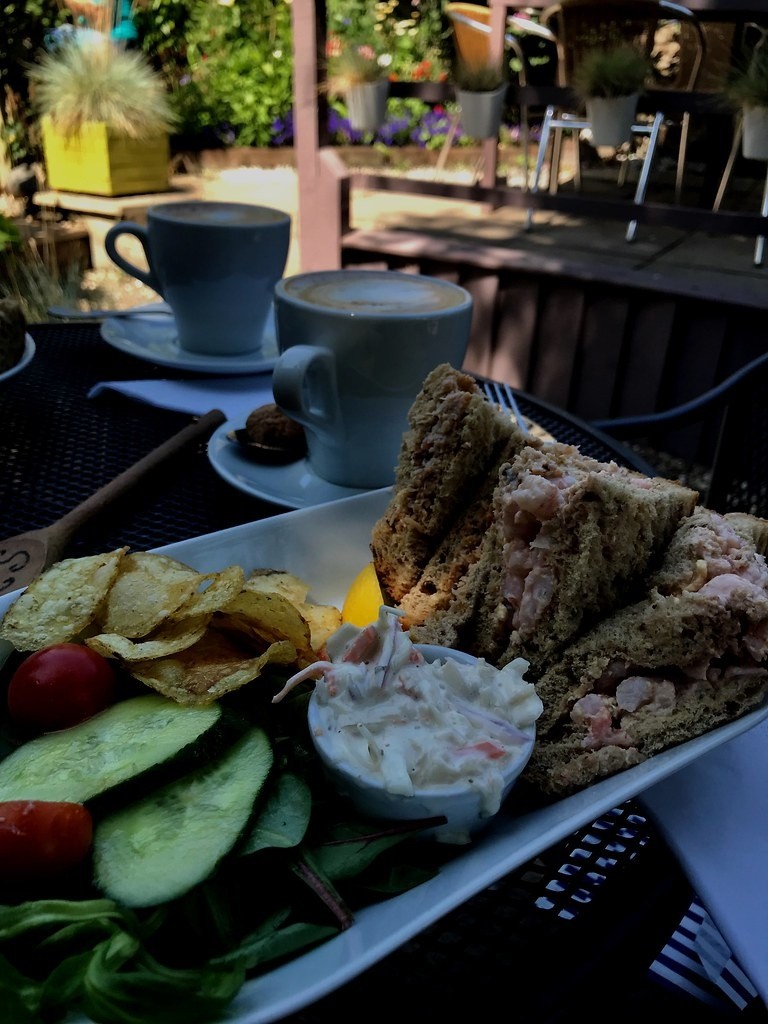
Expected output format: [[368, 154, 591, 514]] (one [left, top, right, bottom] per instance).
[[2, 545, 343, 703]]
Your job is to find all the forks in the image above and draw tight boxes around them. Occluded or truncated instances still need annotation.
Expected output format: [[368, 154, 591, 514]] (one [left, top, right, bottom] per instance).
[[479, 382, 529, 435]]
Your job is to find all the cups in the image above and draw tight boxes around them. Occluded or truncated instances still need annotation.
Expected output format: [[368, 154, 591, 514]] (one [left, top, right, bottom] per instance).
[[271, 270, 473, 487], [106, 203, 291, 352]]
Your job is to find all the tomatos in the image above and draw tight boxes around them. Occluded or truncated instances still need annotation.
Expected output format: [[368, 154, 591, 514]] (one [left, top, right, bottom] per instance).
[[11, 644, 113, 732], [0, 800, 92, 890]]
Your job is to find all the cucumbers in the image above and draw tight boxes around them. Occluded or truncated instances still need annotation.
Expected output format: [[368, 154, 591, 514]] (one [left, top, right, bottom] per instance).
[[92, 727, 278, 910], [0, 692, 242, 810]]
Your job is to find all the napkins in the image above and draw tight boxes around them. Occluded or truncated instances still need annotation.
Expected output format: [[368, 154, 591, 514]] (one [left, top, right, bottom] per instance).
[[635, 715, 768, 1003], [82, 374, 276, 423]]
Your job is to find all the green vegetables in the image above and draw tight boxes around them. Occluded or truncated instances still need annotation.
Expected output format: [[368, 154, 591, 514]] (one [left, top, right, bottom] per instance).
[[0, 817, 447, 1024]]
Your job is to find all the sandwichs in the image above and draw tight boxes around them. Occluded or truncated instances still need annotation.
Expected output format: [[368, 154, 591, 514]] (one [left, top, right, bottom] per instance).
[[371, 362, 768, 790]]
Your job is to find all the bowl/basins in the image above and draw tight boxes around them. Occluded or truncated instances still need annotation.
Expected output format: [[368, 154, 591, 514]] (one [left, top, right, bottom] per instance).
[[308, 645, 536, 832]]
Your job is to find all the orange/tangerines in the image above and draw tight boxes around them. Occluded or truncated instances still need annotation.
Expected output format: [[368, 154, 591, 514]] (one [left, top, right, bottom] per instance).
[[343, 562, 383, 628]]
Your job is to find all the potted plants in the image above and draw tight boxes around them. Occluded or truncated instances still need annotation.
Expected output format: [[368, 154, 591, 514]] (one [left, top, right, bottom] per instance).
[[451, 59, 510, 139], [571, 42, 649, 148], [26, 31, 182, 196], [710, 57, 768, 160], [318, 43, 389, 132]]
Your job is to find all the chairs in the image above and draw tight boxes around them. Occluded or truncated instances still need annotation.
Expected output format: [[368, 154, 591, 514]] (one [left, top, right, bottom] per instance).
[[586, 353, 766, 516], [434, 0, 768, 266]]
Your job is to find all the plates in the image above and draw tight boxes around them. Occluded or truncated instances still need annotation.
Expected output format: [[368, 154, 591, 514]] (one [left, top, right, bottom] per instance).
[[102, 303, 278, 374], [207, 420, 376, 509], [1, 485, 768, 1024], [0, 332, 36, 381]]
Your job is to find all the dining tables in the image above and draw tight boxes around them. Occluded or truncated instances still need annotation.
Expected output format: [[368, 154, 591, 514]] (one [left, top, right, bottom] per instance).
[[0, 320, 768, 1023]]
